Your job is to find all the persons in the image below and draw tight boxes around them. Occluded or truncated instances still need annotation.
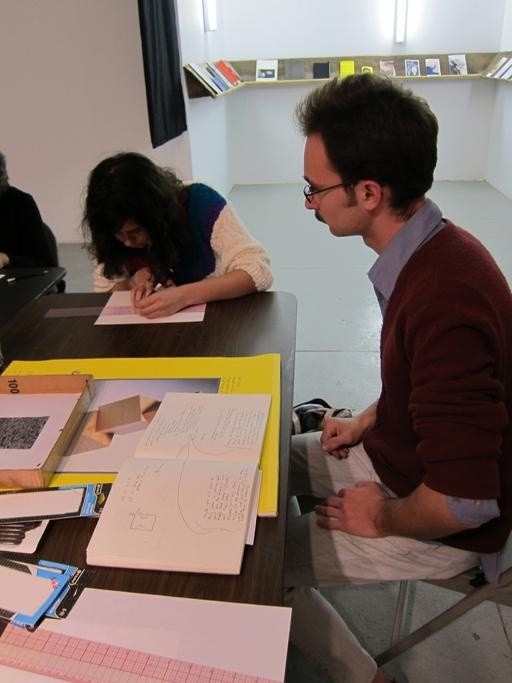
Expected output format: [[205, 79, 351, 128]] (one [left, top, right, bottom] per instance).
[[282, 75, 512, 682], [81, 152, 272, 320], [1, 154, 66, 293]]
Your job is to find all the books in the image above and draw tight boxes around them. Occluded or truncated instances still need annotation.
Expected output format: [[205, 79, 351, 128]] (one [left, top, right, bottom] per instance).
[[87, 392, 273, 576], [1, 373, 96, 489]]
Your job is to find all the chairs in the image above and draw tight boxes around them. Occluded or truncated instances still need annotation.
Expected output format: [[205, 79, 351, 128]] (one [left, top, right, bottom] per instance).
[[350, 543, 512, 668]]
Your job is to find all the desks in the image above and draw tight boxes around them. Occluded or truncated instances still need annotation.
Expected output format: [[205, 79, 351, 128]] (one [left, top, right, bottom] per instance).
[[0, 261, 69, 333], [0, 284, 299, 683]]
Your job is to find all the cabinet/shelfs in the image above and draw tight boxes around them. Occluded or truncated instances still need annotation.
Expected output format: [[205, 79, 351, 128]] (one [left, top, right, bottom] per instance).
[[176, 50, 512, 102]]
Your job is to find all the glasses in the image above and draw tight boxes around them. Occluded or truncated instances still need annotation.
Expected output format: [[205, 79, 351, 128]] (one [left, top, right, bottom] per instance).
[[303, 183, 344, 203]]
